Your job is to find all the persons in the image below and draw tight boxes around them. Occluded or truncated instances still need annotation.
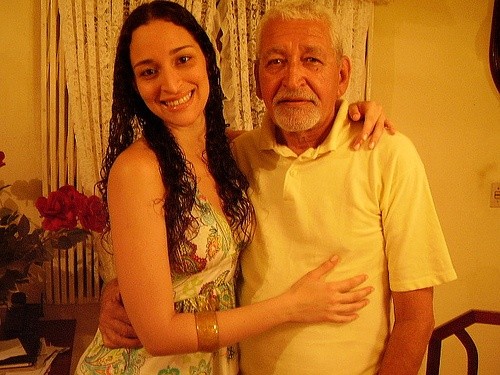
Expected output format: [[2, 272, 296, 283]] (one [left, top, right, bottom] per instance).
[[4, 291, 45, 368], [72, 0, 376, 375], [97, 0, 460, 375]]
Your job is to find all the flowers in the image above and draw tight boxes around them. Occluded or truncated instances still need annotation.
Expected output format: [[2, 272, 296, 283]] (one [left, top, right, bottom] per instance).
[[1, 152, 109, 306]]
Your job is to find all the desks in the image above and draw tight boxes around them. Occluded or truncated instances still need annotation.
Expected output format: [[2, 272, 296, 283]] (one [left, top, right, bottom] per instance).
[[0, 299, 104, 375]]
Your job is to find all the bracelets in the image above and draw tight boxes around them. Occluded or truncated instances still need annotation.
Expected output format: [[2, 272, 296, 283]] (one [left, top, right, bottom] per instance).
[[194, 310, 220, 353]]
